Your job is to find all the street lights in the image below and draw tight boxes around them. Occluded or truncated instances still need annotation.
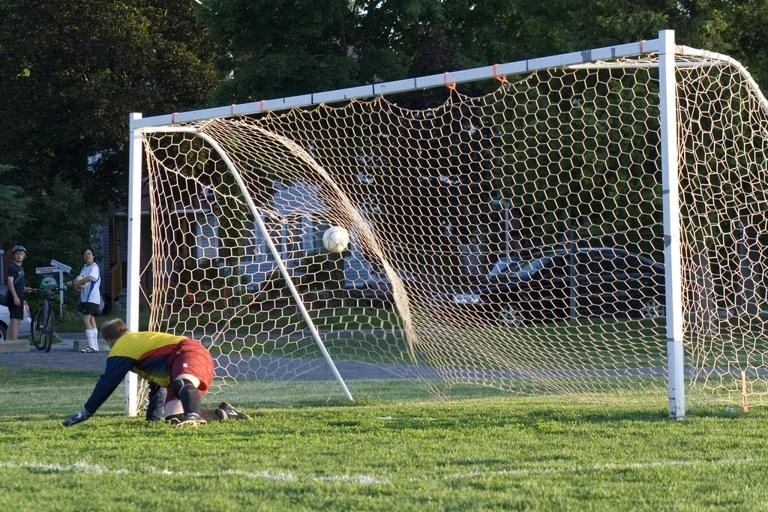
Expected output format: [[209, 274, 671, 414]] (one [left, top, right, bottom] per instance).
[[490, 199, 511, 262]]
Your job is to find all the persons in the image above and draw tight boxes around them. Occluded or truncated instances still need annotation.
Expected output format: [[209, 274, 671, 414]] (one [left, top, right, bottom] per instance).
[[62, 318, 252, 429], [73, 246, 101, 353], [5, 245, 32, 340]]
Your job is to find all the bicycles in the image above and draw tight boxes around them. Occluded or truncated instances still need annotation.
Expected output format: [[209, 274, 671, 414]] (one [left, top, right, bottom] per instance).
[[27, 285, 65, 350]]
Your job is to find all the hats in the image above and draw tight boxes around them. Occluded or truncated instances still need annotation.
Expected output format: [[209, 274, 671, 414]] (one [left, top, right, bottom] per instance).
[[102, 319, 128, 340], [12, 244, 27, 253]]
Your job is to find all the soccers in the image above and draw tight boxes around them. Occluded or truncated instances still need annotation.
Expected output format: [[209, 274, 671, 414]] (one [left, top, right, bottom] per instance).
[[322, 227, 349, 253]]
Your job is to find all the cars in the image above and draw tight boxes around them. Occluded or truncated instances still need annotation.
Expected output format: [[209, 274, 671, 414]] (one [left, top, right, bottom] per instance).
[[443, 247, 666, 328], [0, 304, 34, 341]]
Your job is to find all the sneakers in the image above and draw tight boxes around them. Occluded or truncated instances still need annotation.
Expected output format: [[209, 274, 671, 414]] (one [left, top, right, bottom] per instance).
[[177, 413, 206, 425], [82, 346, 98, 354], [217, 401, 250, 422]]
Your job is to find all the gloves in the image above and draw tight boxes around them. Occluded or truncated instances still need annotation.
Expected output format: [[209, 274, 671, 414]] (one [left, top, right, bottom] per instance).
[[61, 408, 90, 426]]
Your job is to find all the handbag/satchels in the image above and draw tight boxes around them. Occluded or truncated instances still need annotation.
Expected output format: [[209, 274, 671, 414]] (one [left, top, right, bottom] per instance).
[[0, 284, 9, 305]]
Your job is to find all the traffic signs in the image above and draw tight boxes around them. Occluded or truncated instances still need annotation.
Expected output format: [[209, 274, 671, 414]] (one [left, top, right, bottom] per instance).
[[50, 259, 71, 273], [36, 266, 63, 273]]
